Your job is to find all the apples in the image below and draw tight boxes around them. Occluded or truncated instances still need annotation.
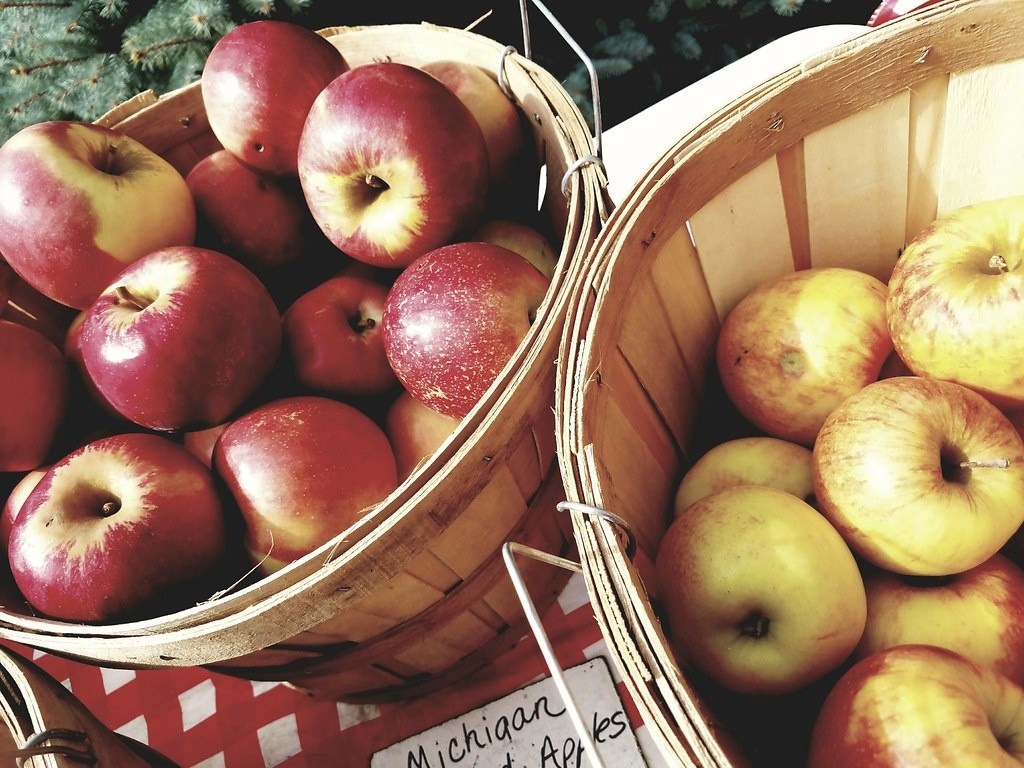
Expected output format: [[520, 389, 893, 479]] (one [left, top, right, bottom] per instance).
[[1, 17, 554, 626], [649, 192, 1024, 768]]
